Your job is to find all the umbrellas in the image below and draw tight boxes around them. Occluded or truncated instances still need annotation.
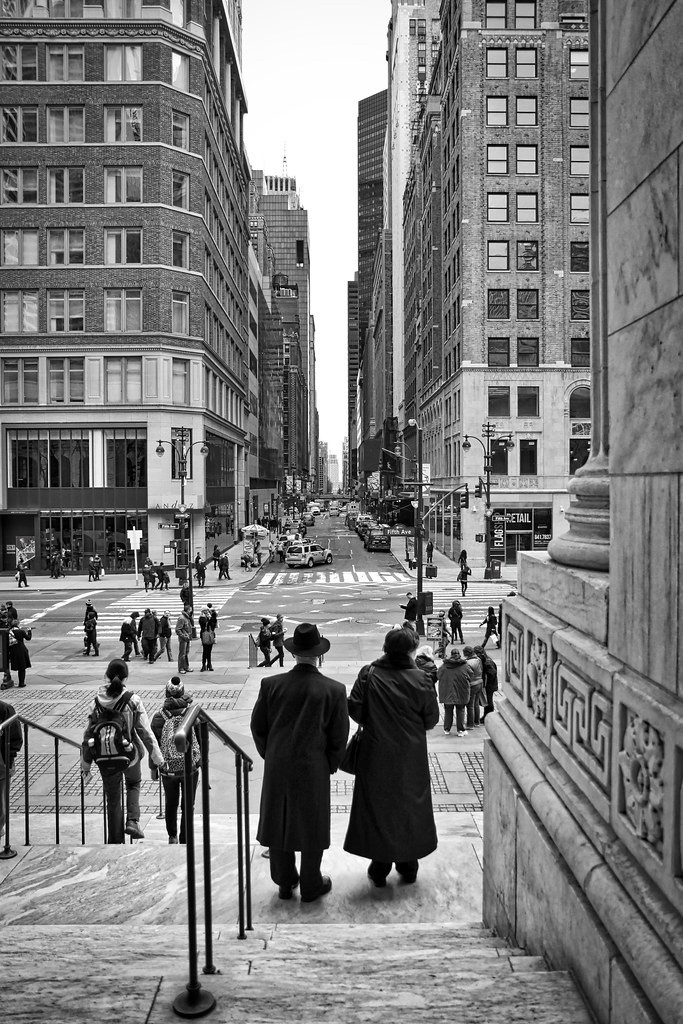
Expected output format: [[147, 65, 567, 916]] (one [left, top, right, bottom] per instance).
[[241, 525, 267, 543]]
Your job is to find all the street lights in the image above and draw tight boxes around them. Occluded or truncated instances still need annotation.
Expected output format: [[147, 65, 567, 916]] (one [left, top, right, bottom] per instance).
[[156, 425, 210, 586], [461, 420, 515, 579], [394, 418, 433, 636]]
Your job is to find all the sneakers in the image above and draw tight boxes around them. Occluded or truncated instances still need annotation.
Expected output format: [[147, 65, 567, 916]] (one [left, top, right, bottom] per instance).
[[126, 822, 144, 839], [444, 723, 484, 737]]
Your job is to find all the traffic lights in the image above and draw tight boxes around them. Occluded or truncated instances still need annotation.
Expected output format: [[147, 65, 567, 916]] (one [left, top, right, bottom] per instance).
[[461, 491, 467, 507], [474, 484, 481, 499]]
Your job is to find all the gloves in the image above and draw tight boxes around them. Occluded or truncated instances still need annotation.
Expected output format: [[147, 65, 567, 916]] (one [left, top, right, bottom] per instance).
[[151, 769, 158, 781], [81, 771, 91, 779], [160, 762, 170, 776]]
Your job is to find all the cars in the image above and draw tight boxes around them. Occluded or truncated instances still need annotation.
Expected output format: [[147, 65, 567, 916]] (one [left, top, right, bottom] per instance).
[[346, 511, 380, 539], [329, 506, 339, 517], [278, 512, 314, 548], [311, 506, 321, 514]]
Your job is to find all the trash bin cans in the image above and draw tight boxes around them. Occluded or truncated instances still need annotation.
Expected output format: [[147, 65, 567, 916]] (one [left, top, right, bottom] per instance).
[[491, 559, 501, 579]]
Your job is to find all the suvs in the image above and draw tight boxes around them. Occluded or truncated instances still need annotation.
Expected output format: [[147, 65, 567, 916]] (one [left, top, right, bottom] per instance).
[[364, 526, 391, 551], [285, 544, 333, 568]]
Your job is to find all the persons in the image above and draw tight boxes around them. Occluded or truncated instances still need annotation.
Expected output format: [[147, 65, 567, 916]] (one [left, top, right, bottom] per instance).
[[0, 546, 308, 844], [206, 518, 233, 539], [251, 623, 348, 902], [258, 516, 309, 563], [44, 542, 128, 570], [340, 630, 440, 887], [398, 542, 516, 736]]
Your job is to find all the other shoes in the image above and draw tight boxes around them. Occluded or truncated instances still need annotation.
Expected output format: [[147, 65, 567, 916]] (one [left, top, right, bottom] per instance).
[[450, 639, 465, 644], [368, 866, 386, 887], [169, 836, 178, 844], [121, 654, 172, 664], [179, 668, 193, 673], [402, 873, 416, 883]]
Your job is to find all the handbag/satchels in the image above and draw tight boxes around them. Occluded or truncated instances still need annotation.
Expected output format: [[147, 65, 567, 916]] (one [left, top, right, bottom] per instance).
[[339, 725, 362, 775], [202, 622, 214, 644], [490, 632, 498, 644], [458, 570, 462, 579], [479, 688, 488, 706], [84, 636, 88, 647]]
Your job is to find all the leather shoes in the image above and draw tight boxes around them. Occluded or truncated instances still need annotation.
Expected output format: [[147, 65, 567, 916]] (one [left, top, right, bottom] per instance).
[[279, 887, 293, 899], [300, 876, 332, 902]]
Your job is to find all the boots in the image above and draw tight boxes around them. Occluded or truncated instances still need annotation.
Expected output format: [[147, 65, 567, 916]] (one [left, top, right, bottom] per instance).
[[19, 678, 27, 688], [83, 649, 99, 657], [200, 658, 213, 672]]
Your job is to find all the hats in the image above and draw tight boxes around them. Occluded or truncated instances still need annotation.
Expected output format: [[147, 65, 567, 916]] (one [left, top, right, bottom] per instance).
[[284, 623, 330, 657], [261, 614, 284, 627], [144, 608, 150, 612], [86, 599, 95, 620], [166, 677, 184, 698]]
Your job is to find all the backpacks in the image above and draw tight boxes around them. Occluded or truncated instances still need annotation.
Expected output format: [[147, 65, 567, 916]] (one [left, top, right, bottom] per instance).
[[160, 707, 201, 777], [88, 691, 139, 775], [255, 633, 260, 647]]
